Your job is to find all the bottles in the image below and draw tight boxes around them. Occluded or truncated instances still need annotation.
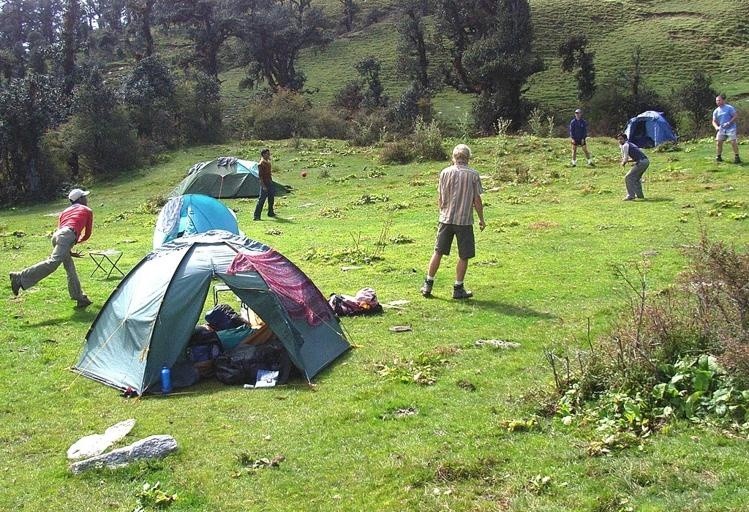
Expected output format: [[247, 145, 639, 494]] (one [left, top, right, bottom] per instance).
[[159, 364, 172, 394]]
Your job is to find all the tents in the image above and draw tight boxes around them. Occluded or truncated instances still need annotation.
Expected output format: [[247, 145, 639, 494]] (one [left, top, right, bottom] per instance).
[[622, 110, 680, 149], [149, 192, 241, 246], [69, 229, 358, 400], [164, 156, 291, 199]]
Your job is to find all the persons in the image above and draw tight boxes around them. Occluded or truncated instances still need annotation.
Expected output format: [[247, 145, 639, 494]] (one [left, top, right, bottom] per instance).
[[711, 92, 742, 164], [253, 149, 278, 220], [616, 132, 650, 201], [9, 188, 94, 307], [419, 143, 487, 300], [570, 109, 595, 168]]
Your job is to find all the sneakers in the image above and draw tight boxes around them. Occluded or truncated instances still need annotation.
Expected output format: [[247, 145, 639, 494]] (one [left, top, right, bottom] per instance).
[[587, 159, 592, 165], [76, 299, 92, 307], [715, 155, 722, 161], [8, 271, 20, 296], [734, 155, 739, 163], [571, 160, 576, 167]]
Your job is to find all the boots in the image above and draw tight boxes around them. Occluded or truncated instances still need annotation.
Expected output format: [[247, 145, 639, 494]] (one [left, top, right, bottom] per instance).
[[452, 283, 473, 299], [420, 279, 433, 297]]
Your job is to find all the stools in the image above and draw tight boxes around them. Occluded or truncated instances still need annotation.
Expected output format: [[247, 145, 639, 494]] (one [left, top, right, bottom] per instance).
[[89, 251, 125, 281], [210, 282, 235, 308]]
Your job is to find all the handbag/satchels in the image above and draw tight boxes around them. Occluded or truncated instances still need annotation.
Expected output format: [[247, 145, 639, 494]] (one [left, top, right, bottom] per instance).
[[325, 286, 382, 317]]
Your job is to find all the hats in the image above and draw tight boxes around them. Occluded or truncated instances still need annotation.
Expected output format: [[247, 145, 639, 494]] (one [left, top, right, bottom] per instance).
[[574, 109, 581, 113], [68, 189, 90, 203]]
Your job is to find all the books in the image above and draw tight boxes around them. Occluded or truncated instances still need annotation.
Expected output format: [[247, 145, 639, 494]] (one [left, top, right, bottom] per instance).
[[254, 365, 279, 390]]
